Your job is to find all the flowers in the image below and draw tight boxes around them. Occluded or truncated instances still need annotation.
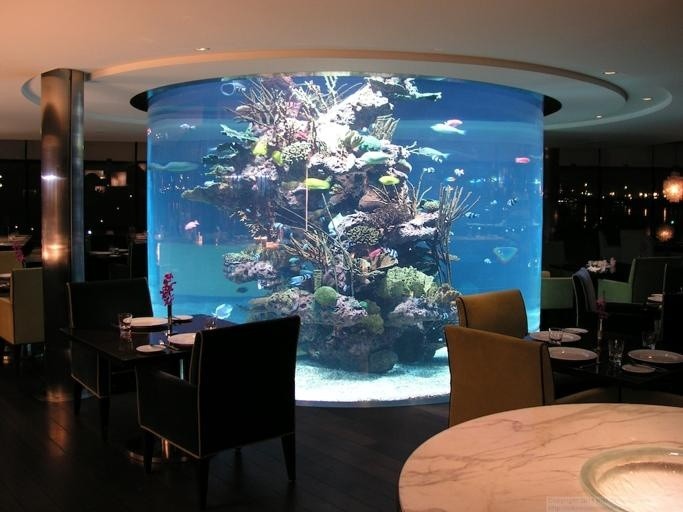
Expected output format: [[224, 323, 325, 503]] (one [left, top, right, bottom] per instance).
[[13, 241, 26, 264], [593, 295, 609, 319], [586, 256, 616, 275], [160, 272, 176, 307]]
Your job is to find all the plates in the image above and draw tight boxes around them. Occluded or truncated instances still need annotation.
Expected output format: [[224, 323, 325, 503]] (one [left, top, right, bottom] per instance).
[[531, 330, 582, 346], [173, 315, 192, 322], [136, 344, 165, 354], [580, 446, 683, 511], [168, 332, 196, 345], [627, 348, 682, 364], [620, 362, 654, 374], [130, 316, 168, 327], [565, 325, 588, 335], [546, 345, 597, 363]]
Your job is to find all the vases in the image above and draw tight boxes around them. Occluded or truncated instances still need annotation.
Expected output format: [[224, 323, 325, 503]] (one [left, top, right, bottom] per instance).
[[165, 302, 178, 335], [596, 317, 604, 364]]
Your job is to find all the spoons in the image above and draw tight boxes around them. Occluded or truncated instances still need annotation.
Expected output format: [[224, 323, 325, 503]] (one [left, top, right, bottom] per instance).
[[158, 339, 179, 351]]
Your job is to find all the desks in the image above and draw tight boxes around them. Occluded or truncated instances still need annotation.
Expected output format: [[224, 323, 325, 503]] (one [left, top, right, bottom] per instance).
[[523, 331, 683, 391]]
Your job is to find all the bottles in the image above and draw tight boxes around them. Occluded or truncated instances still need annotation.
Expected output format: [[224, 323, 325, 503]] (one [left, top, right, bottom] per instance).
[[654, 305, 663, 339]]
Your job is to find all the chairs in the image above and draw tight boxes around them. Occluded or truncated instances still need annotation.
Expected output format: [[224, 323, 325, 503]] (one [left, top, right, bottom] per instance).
[[572, 268, 661, 345], [60, 278, 154, 440], [135, 315, 301, 512], [444, 326, 607, 431], [455, 289, 529, 340], [0, 267, 45, 374], [542, 270, 573, 309], [0, 251, 24, 273], [598, 256, 682, 304]]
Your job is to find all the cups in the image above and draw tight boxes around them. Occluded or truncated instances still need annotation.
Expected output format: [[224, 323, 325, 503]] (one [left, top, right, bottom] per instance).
[[641, 330, 658, 349], [118, 311, 133, 331], [548, 326, 564, 343], [206, 317, 216, 330], [607, 335, 624, 363], [592, 313, 609, 343]]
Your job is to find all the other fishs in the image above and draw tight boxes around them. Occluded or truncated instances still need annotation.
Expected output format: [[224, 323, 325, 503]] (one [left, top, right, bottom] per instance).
[[215, 117, 468, 287], [502, 196, 519, 211], [185, 220, 199, 231], [147, 161, 163, 172], [465, 212, 481, 219], [515, 157, 530, 163], [163, 160, 202, 174], [214, 303, 233, 320]]
